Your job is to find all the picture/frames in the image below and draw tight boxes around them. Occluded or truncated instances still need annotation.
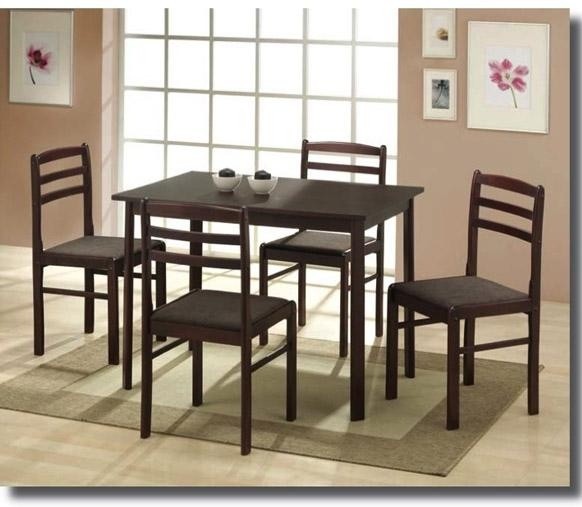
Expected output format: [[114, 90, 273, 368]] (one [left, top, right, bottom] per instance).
[[6, 10, 75, 109], [422, 9, 550, 134]]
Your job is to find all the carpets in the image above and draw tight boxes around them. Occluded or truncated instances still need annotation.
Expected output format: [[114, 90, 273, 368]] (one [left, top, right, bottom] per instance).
[[0, 315, 545, 478]]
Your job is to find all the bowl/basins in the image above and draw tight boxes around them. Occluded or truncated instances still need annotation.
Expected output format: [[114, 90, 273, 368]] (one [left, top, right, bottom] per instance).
[[212, 174, 242, 192], [247, 175, 279, 195]]
